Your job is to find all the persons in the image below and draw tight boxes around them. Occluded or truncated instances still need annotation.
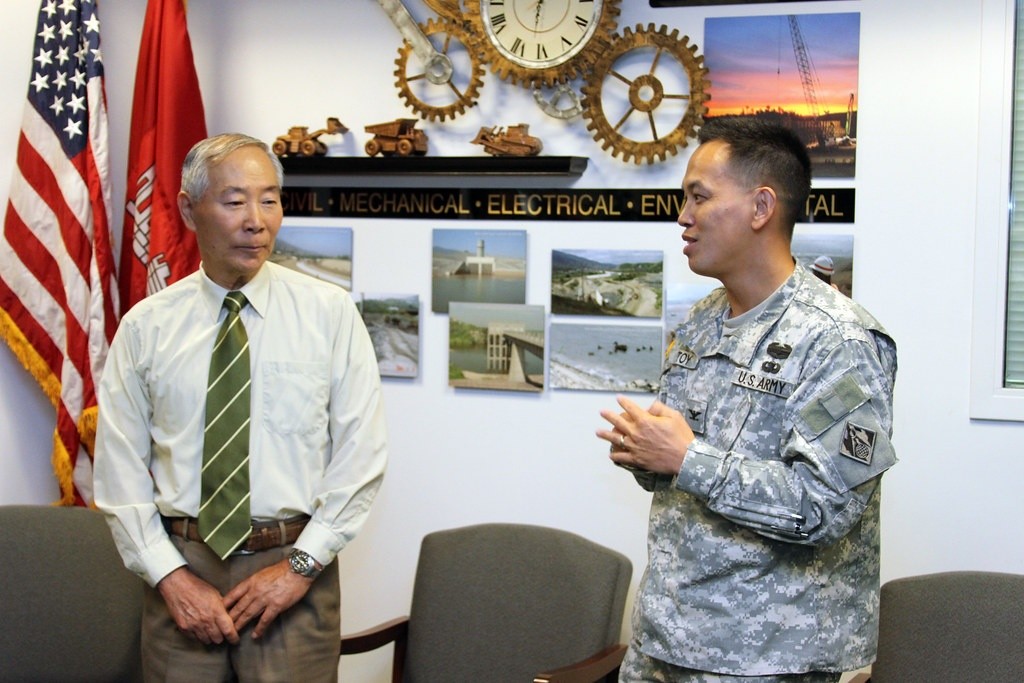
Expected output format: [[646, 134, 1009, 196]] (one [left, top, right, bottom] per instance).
[[808, 256, 834, 286], [94, 134, 387, 683], [595, 116, 898, 683]]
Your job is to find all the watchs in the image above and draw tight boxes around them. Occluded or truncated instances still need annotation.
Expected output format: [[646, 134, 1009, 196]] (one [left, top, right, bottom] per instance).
[[288, 547, 322, 580]]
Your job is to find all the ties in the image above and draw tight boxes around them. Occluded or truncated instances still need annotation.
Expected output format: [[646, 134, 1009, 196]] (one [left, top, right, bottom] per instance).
[[197, 292, 252, 561]]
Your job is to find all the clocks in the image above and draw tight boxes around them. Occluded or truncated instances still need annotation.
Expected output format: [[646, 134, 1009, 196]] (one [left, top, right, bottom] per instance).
[[462, 0, 621, 89]]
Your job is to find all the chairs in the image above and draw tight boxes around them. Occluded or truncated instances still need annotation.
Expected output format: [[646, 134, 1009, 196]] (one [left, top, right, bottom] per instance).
[[0, 504, 1024, 683]]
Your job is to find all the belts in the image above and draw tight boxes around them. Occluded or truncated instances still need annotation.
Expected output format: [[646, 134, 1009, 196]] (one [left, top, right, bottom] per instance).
[[160, 514, 312, 557]]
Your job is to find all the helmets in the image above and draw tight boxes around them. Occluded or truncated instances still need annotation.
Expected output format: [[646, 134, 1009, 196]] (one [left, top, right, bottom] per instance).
[[809, 256, 835, 277]]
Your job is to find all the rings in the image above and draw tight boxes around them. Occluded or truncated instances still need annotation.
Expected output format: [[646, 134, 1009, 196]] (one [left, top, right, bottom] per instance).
[[621, 435, 626, 449]]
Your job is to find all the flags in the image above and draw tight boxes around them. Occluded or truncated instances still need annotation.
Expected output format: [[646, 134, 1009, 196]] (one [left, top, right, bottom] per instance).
[[0, 0, 117, 509], [119, 0, 211, 318]]
